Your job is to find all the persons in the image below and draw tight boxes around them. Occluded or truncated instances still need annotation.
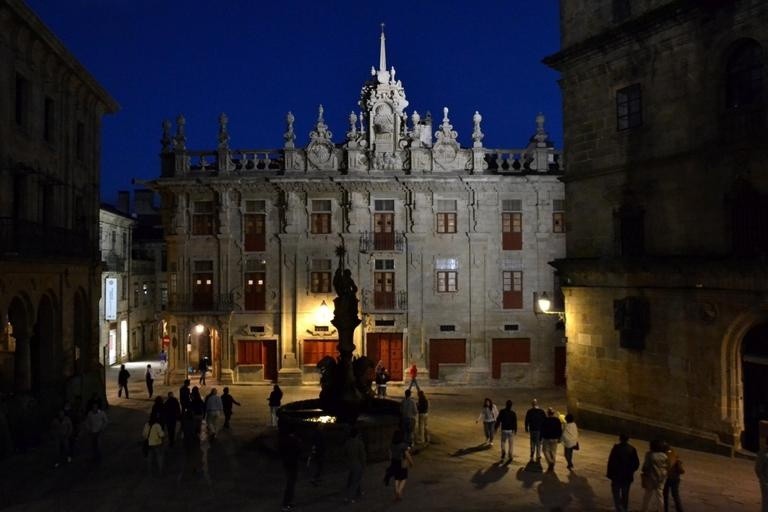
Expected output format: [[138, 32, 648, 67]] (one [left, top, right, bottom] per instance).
[[62, 399, 83, 448], [389, 430, 416, 500], [345, 429, 368, 503], [118, 364, 131, 399], [375, 366, 390, 396], [198, 355, 209, 385], [84, 392, 109, 453], [146, 364, 155, 398], [408, 364, 422, 391], [159, 350, 167, 360], [268, 384, 285, 428], [142, 379, 241, 457], [307, 443, 323, 488], [281, 435, 300, 507], [401, 389, 430, 448], [475, 397, 684, 511]]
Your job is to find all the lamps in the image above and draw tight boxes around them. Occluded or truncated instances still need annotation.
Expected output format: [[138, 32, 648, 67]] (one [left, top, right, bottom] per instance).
[[534, 291, 565, 320]]
